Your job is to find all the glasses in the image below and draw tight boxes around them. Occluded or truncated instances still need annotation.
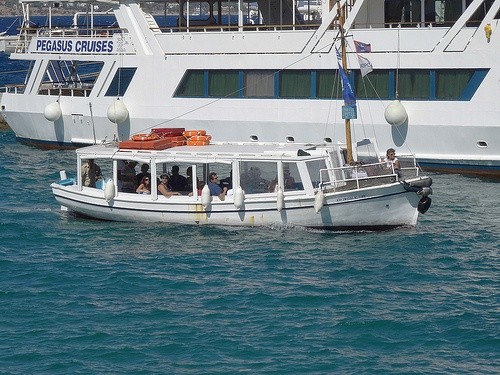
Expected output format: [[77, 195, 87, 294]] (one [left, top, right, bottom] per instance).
[[145, 179, 149, 181], [163, 178, 168, 179], [171, 170, 174, 171], [215, 177, 218, 179], [391, 153, 395, 156]]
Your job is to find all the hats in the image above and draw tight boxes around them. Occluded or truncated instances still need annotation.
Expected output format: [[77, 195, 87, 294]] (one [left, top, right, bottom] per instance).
[[160, 173, 170, 178]]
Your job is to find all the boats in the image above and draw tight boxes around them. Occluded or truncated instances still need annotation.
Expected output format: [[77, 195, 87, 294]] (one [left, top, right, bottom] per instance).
[[49, 129, 434, 228], [0, 0, 496, 177]]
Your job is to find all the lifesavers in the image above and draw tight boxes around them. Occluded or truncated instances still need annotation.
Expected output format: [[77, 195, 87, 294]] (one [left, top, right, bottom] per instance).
[[131, 134, 159, 141], [191, 135, 211, 141], [183, 130, 207, 137], [187, 141, 210, 146]]
